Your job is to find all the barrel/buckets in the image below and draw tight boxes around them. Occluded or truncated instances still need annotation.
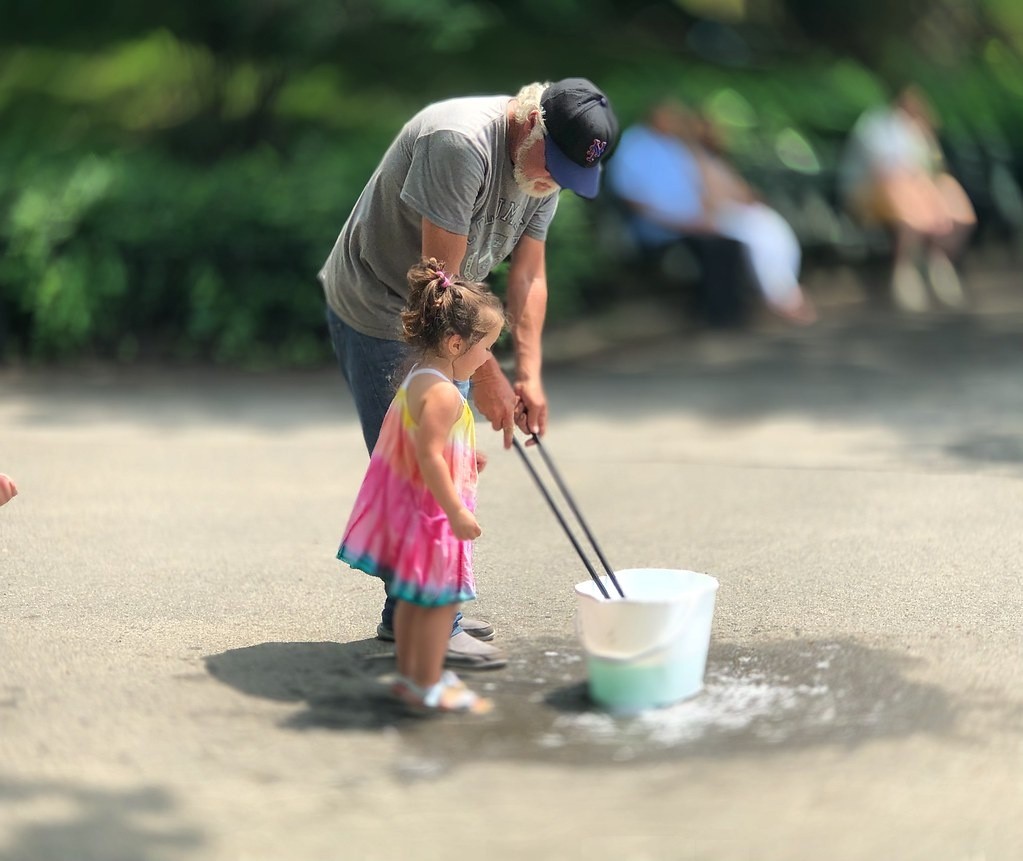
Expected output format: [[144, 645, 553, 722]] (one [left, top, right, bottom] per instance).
[[575, 568, 720, 709]]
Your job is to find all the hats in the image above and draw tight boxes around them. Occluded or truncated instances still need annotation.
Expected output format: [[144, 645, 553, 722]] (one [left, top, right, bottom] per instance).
[[540, 78, 617, 200]]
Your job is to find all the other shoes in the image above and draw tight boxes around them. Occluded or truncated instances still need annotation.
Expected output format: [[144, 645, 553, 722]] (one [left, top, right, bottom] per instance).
[[375, 612, 496, 640], [444, 629, 509, 670]]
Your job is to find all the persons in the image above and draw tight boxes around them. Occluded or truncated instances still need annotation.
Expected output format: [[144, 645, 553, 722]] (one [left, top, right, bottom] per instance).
[[841, 78, 978, 310], [319, 78, 618, 670], [604, 94, 821, 364], [336, 256, 512, 716]]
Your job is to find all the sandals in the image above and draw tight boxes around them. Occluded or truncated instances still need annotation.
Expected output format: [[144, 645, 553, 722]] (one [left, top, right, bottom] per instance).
[[381, 668, 484, 715]]
